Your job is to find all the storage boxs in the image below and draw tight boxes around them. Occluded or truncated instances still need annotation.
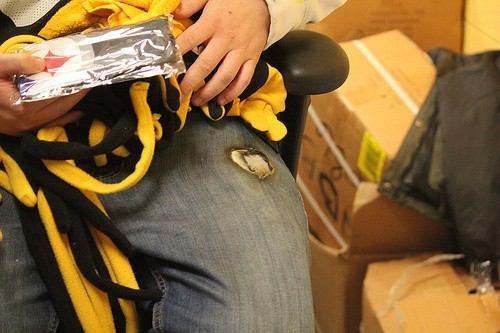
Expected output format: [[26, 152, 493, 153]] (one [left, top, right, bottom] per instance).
[[306, 231, 424, 333], [360, 253, 500, 332], [297, 27, 445, 259], [306, 0, 466, 57]]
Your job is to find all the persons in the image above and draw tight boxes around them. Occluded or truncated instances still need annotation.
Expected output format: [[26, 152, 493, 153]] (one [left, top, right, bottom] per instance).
[[0, 0, 347, 333]]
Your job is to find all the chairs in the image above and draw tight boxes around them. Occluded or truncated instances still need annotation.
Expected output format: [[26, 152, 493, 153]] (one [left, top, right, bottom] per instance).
[[264, 27, 350, 179]]
